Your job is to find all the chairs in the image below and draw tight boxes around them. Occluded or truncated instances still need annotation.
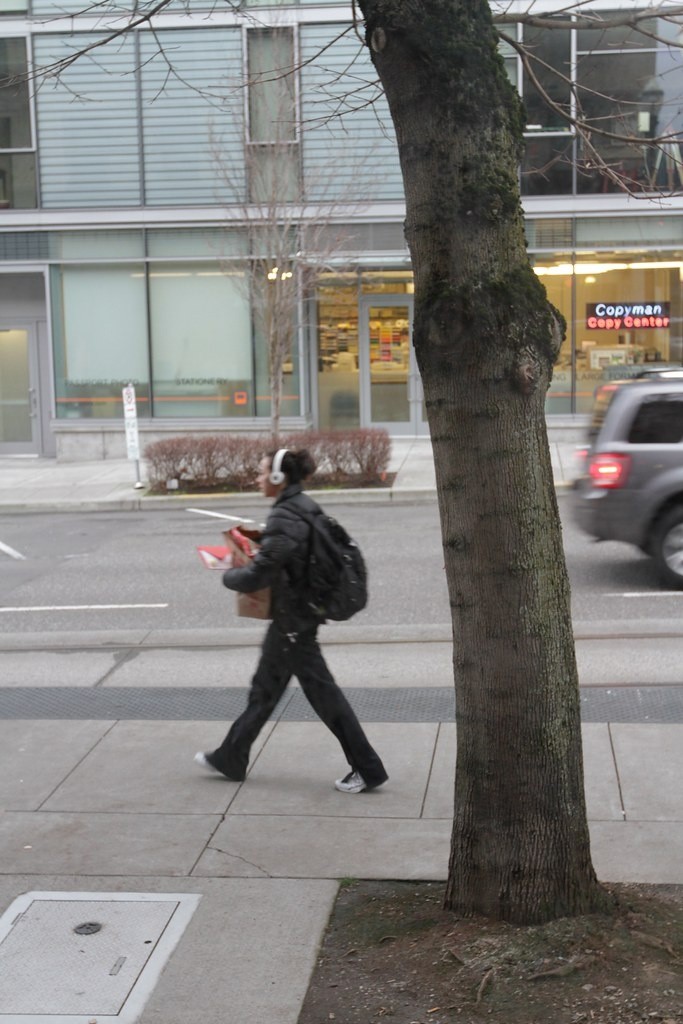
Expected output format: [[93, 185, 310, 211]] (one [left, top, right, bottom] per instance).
[[603, 142, 642, 193]]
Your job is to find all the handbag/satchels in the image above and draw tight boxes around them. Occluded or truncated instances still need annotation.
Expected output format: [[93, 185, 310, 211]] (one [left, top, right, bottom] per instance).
[[222, 524, 273, 620]]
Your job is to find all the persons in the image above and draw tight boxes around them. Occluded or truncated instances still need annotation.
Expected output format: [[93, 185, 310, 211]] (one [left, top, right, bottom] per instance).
[[193, 449, 390, 794]]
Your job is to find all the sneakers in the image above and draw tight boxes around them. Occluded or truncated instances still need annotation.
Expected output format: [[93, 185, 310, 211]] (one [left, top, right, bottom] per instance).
[[194, 752, 217, 771], [335, 771, 367, 794]]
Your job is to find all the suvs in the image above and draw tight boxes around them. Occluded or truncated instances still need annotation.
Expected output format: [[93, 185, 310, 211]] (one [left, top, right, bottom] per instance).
[[569, 356, 682, 584]]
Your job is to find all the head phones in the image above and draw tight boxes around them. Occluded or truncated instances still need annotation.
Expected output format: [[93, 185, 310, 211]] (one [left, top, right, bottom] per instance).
[[269, 449, 288, 485]]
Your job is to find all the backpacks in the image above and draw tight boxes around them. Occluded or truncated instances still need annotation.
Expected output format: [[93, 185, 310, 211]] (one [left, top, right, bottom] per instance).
[[277, 501, 367, 621]]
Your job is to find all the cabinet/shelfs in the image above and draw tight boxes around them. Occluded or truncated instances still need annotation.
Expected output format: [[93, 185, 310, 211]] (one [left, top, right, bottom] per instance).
[[319, 316, 410, 371]]
